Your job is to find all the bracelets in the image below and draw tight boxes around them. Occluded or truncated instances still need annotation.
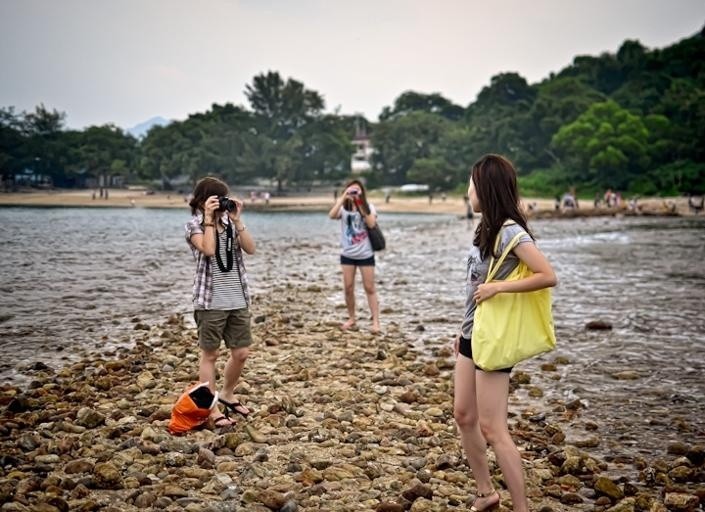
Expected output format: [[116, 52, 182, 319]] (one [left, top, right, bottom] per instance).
[[202, 223, 214, 227], [235, 226, 246, 232], [355, 199, 362, 207]]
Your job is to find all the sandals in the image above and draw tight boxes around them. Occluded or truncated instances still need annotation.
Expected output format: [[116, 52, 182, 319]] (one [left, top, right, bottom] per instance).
[[466, 490, 501, 512]]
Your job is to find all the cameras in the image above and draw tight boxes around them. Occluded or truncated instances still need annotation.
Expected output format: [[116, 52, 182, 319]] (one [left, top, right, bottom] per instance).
[[216, 195, 236, 212], [348, 189, 358, 197]]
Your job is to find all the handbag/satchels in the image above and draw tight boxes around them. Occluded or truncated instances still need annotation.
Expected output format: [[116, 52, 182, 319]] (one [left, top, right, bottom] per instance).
[[362, 221, 387, 253], [165, 379, 222, 436], [469, 254, 562, 377]]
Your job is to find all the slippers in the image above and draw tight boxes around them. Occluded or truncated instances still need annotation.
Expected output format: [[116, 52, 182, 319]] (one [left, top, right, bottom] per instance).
[[217, 391, 252, 419], [210, 412, 237, 428]]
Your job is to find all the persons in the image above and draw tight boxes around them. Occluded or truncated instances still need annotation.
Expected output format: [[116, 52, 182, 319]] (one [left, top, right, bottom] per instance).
[[463, 197, 474, 227], [328, 179, 381, 335], [453, 153, 558, 512], [183, 176, 256, 428], [249, 190, 270, 206], [517, 185, 677, 217]]
